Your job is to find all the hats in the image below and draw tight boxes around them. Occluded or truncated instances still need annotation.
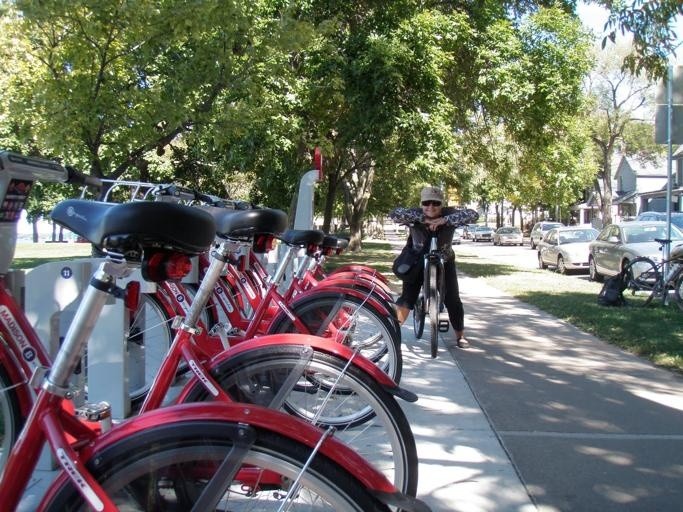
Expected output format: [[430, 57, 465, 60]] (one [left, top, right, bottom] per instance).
[[421, 188, 443, 203]]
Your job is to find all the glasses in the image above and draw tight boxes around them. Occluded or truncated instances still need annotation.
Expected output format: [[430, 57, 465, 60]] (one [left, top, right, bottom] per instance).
[[421, 201, 441, 206]]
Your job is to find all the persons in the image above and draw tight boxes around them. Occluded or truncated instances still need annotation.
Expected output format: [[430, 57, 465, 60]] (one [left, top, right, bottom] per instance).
[[390, 187, 479, 349]]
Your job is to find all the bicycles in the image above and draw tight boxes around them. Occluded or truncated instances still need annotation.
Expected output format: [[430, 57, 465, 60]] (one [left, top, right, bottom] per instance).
[[394, 215, 469, 358], [619, 238, 683, 311]]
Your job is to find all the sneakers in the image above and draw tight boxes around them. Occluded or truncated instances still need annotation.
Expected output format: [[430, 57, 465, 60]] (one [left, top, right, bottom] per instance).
[[457, 337, 469, 348]]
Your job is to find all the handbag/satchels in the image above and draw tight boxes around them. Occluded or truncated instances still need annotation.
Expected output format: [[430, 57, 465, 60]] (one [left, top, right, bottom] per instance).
[[392, 247, 425, 285]]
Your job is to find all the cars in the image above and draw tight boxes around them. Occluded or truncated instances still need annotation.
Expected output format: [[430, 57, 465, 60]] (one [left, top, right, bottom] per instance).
[[530, 211, 683, 291], [451, 224, 523, 246]]
